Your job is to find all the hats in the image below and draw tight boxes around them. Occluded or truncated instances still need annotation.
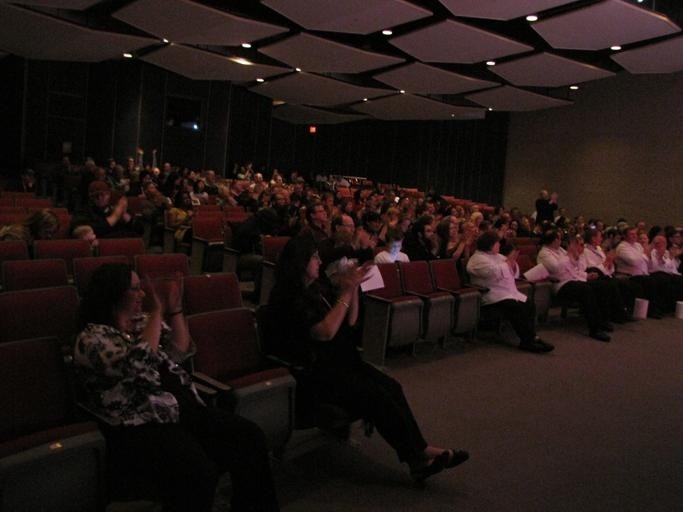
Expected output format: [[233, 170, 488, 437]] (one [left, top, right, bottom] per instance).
[[89, 180, 113, 192]]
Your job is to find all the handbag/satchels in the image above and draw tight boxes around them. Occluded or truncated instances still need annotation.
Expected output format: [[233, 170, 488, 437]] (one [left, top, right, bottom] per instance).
[[168, 207, 187, 227]]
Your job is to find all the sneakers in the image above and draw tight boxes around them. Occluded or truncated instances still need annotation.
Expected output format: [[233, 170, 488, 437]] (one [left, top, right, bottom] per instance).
[[517, 336, 556, 354], [581, 319, 615, 342]]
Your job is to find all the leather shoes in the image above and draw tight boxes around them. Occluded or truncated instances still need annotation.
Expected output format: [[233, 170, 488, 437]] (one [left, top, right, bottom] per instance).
[[649, 308, 665, 319], [412, 447, 470, 482]]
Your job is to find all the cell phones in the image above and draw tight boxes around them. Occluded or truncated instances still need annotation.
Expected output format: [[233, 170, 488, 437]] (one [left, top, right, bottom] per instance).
[[394, 260, 401, 263]]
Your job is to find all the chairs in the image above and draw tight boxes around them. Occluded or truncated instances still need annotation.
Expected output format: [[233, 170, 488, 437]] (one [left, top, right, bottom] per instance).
[[1, 365, 394, 511], [0, 166, 681, 364]]
